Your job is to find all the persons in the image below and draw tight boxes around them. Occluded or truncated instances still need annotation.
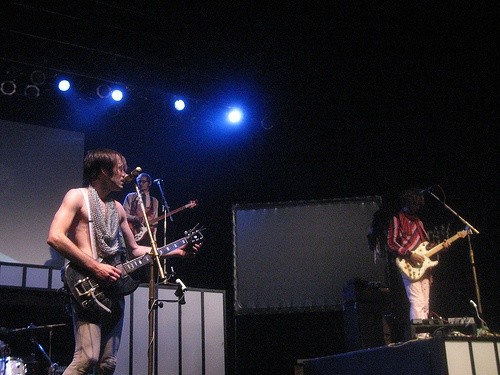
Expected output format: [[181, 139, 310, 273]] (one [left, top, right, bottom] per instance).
[[386, 189, 452, 339], [47, 147, 203, 375], [122, 172, 158, 283]]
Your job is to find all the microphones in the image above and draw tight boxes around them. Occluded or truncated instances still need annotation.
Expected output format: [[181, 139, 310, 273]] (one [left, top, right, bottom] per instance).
[[124, 167, 141, 182], [420, 185, 436, 195]]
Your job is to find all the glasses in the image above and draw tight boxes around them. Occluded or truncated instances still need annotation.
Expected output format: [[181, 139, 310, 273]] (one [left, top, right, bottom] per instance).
[[138, 180, 147, 184]]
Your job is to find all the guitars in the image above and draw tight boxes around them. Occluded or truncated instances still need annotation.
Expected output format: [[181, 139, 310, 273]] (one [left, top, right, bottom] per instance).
[[61, 224, 204, 312], [395, 228, 473, 282], [128, 200, 198, 243]]
